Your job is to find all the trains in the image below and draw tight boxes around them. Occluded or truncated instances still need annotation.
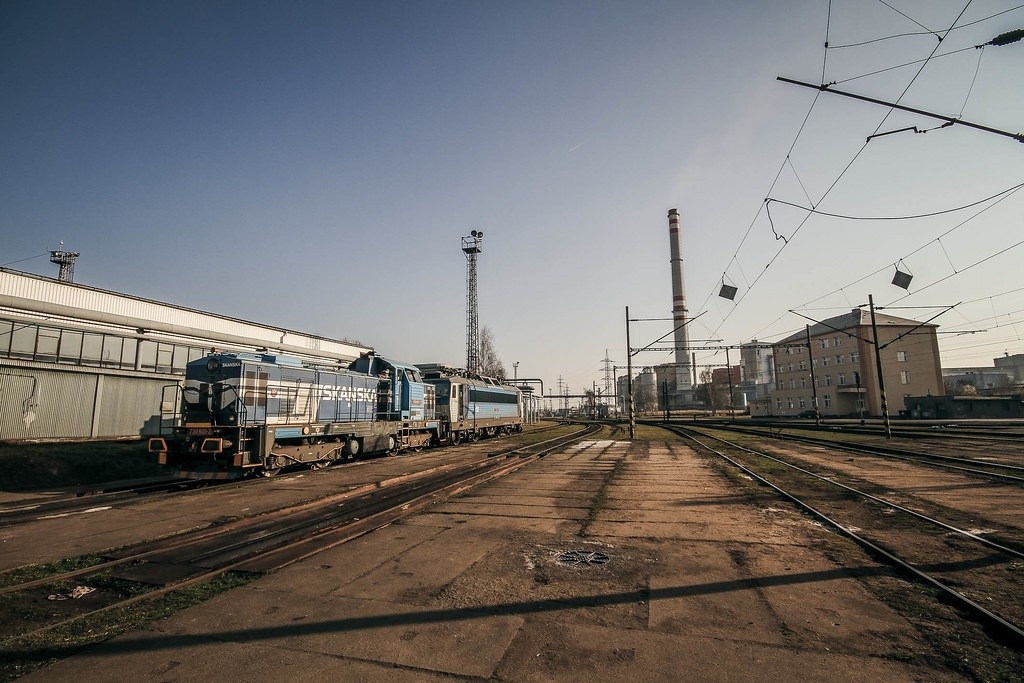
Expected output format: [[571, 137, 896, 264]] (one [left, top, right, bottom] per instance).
[[147, 347, 524, 483]]
